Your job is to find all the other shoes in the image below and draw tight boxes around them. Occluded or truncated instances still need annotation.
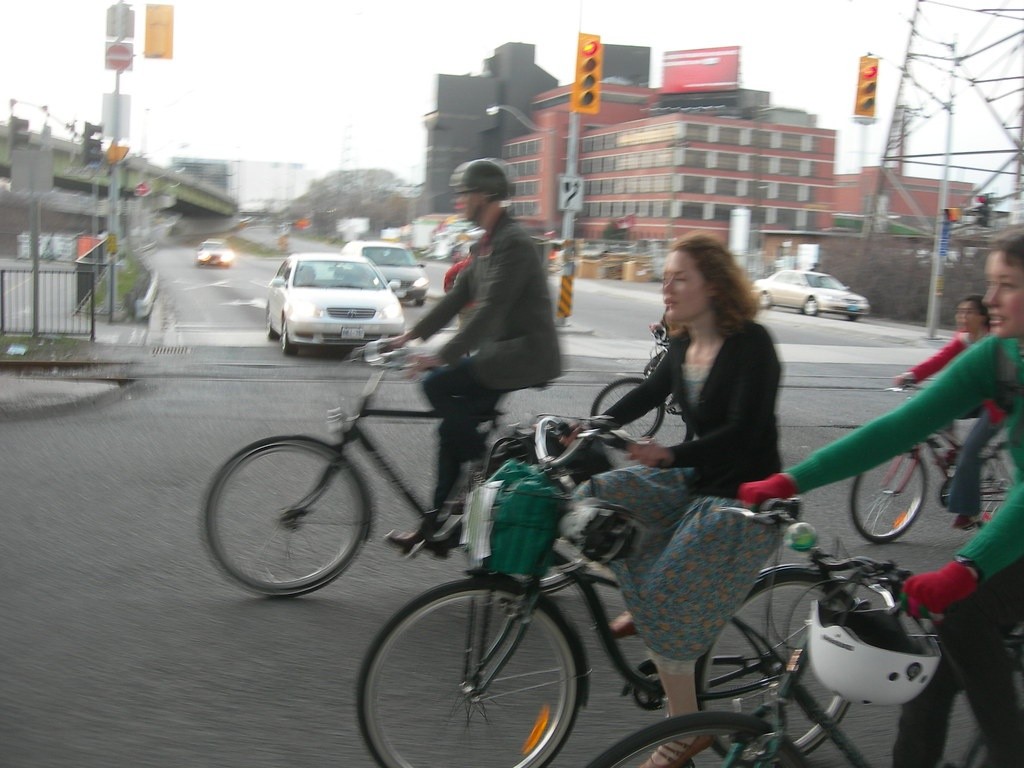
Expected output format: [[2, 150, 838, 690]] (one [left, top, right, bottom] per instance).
[[952, 513, 981, 528], [388, 513, 451, 559], [638, 732, 712, 768], [608, 610, 635, 636]]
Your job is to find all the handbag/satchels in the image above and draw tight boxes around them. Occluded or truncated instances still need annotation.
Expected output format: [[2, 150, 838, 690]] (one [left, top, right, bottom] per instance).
[[483, 417, 614, 484], [479, 457, 566, 573]]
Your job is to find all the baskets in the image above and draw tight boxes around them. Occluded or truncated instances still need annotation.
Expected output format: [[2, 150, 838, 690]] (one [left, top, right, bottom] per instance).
[[464, 464, 571, 574]]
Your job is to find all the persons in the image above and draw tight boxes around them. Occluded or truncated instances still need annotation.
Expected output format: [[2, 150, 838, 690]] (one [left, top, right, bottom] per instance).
[[891, 293, 1004, 528], [559, 231, 782, 767], [651, 301, 685, 338], [444, 241, 477, 328], [365, 157, 564, 562], [738, 229, 1024, 768]]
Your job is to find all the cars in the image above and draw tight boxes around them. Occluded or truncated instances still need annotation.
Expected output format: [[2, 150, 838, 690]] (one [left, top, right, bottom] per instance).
[[195, 238, 234, 269], [265, 253, 406, 355], [341, 240, 429, 306], [753, 269, 871, 321]]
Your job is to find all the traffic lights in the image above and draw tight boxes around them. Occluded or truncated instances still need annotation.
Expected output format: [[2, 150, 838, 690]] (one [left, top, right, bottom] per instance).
[[82, 121, 103, 165], [854, 56, 878, 116], [571, 32, 601, 115], [977, 193, 988, 226]]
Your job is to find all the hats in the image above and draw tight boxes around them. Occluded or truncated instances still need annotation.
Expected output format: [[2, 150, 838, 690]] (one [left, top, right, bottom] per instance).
[[450, 159, 508, 200]]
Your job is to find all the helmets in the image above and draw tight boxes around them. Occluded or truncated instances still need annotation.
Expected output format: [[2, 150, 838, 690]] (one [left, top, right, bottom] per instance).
[[808, 590, 941, 705]]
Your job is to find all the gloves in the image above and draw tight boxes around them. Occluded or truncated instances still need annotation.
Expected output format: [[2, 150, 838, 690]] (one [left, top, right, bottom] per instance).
[[901, 563, 978, 621], [738, 474, 795, 509]]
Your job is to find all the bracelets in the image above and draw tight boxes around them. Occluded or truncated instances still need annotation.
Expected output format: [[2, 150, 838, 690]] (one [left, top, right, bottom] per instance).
[[956, 556, 983, 581]]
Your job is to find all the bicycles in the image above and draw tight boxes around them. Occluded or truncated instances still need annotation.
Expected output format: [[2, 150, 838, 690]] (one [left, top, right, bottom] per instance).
[[589, 322, 687, 439], [850, 380, 1015, 543], [198, 337, 643, 600], [353, 411, 977, 768]]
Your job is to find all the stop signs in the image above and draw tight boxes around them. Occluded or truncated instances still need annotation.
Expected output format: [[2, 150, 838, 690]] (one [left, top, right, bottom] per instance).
[[134, 181, 152, 197], [104, 42, 133, 72]]
